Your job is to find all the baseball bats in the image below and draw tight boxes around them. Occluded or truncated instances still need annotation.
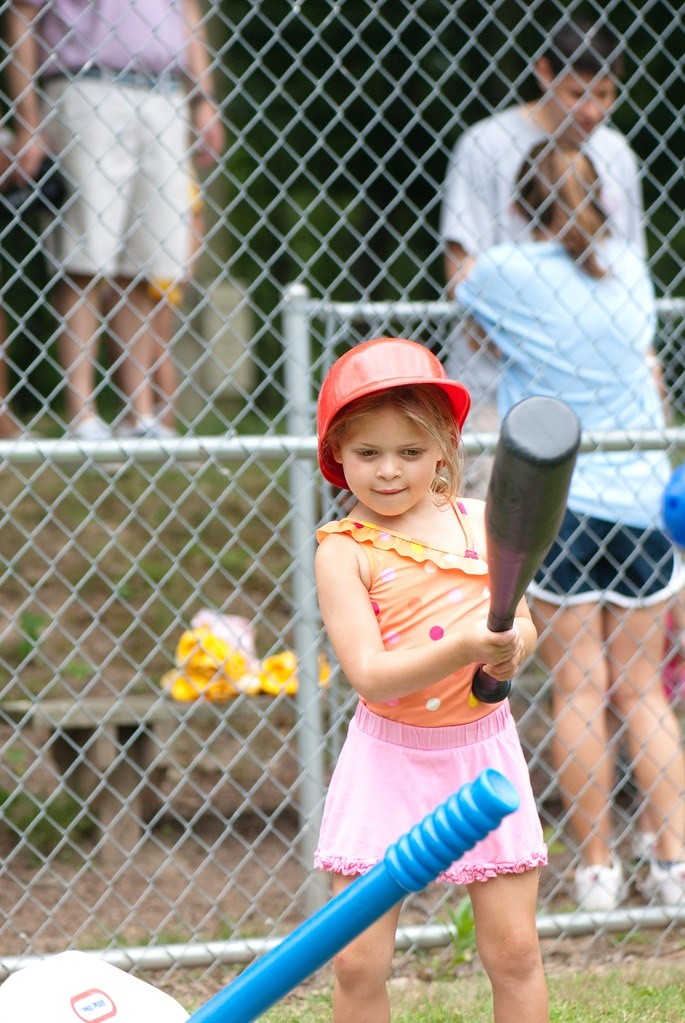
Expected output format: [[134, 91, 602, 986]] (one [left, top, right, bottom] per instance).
[[471, 395, 583, 703]]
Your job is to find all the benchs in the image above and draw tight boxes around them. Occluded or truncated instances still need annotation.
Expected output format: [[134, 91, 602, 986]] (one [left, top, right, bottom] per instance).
[[4, 672, 552, 870]]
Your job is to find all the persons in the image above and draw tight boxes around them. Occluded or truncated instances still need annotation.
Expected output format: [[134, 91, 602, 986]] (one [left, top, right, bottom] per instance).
[[109, 167, 207, 437], [455, 138, 684, 915], [310, 335, 557, 1023], [0, 122, 43, 442], [1, 0, 228, 479], [438, 19, 653, 500]]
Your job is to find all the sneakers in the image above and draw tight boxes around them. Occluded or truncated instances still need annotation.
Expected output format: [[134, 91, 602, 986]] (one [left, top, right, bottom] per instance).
[[571, 858, 623, 914], [633, 855, 685, 908]]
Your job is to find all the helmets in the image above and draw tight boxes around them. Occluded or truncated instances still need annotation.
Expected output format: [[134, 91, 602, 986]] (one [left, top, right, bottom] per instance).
[[315, 338, 470, 489]]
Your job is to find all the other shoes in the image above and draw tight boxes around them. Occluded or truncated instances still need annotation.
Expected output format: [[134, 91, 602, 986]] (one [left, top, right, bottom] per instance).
[[67, 410, 170, 464]]
